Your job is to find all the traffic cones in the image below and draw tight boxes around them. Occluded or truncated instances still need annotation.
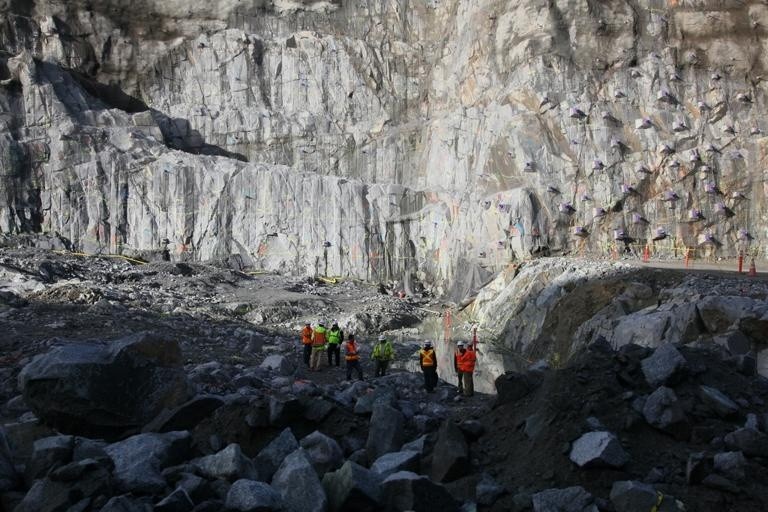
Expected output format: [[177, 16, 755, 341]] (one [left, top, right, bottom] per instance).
[[747, 258, 758, 277]]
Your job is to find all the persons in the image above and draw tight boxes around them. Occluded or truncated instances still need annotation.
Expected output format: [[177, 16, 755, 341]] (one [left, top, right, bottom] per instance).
[[326, 323, 343, 369], [461, 342, 476, 397], [420, 340, 436, 393], [310, 320, 326, 371], [344, 335, 362, 380], [371, 335, 394, 377], [454, 341, 466, 393], [302, 320, 313, 364]]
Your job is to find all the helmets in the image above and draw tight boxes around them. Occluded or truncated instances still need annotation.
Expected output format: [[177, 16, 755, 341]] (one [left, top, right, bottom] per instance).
[[457, 340, 464, 346], [379, 335, 387, 340], [304, 320, 338, 326], [424, 339, 431, 344]]
